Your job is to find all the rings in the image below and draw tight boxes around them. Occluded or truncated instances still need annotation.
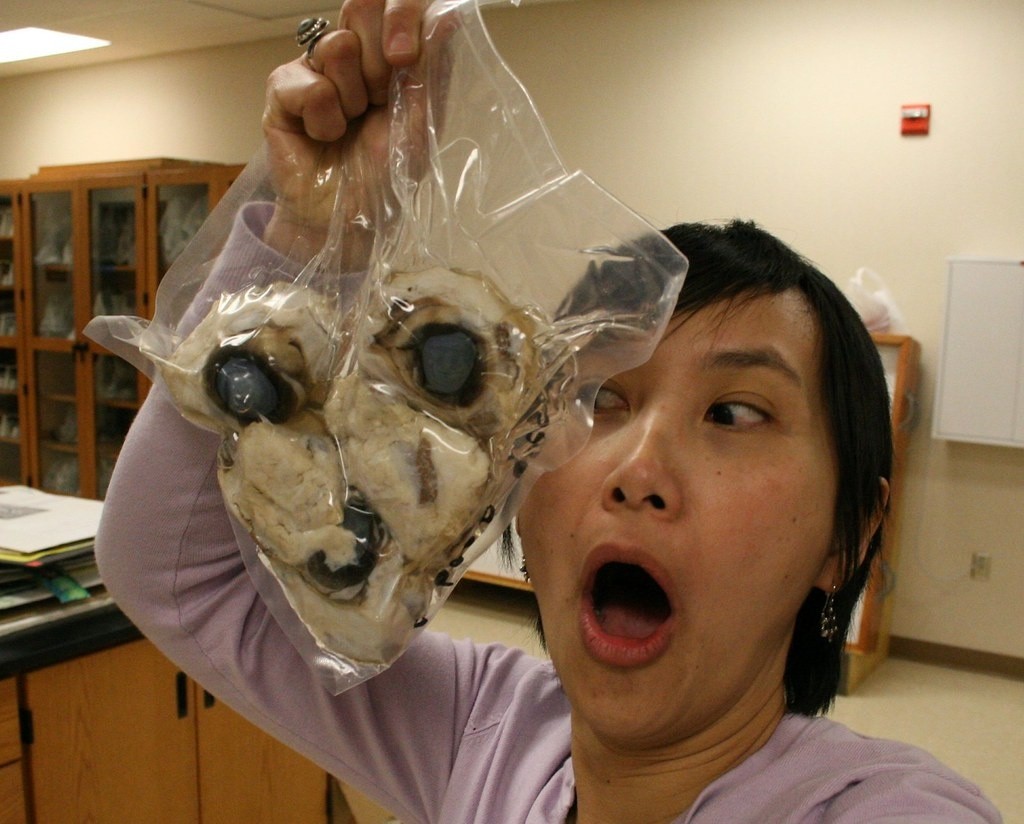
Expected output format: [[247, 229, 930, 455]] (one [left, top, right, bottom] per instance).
[[296, 17, 331, 59]]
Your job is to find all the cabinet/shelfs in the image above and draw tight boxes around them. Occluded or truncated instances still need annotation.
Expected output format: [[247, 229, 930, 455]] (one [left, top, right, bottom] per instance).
[[0, 157, 247, 500], [1, 637, 330, 823]]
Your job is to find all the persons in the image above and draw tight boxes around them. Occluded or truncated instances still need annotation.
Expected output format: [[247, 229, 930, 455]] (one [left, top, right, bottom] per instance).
[[93, 0, 1003, 824]]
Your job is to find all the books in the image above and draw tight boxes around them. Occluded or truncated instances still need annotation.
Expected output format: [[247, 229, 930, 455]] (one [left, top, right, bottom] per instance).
[[1, 485, 103, 566]]
